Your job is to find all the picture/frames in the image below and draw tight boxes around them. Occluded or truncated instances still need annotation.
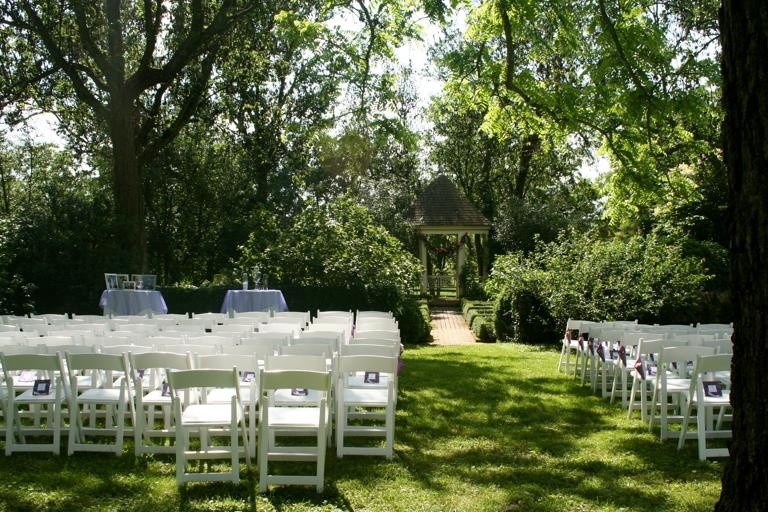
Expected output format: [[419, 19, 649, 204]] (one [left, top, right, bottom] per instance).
[[105, 274, 117, 289], [132, 274, 157, 289], [117, 274, 128, 289], [123, 281, 135, 289]]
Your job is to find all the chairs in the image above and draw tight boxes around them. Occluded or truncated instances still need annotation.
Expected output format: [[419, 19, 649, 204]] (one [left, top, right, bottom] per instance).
[[1, 311, 401, 493], [557, 318, 735, 462]]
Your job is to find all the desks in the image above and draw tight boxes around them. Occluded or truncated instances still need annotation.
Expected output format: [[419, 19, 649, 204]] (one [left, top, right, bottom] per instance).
[[222, 290, 288, 312], [99, 289, 167, 316]]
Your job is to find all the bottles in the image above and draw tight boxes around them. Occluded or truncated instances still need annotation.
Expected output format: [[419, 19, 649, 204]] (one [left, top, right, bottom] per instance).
[[263, 274, 269, 290], [242, 273, 248, 290]]
